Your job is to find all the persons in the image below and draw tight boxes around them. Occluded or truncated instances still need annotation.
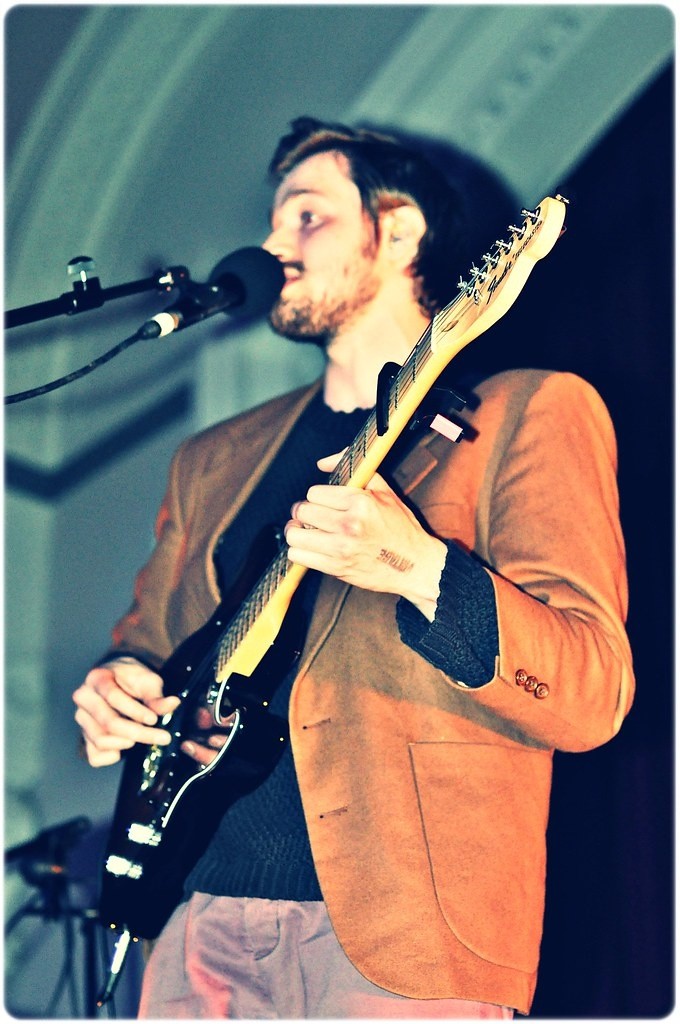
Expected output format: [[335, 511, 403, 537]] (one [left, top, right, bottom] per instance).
[[70, 115, 634, 1020]]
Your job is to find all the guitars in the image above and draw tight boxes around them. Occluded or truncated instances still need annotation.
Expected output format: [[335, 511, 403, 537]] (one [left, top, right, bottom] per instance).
[[95, 193, 571, 942]]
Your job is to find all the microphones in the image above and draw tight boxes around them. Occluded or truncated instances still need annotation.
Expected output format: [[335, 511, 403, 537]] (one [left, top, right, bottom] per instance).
[[137, 244, 288, 339]]
